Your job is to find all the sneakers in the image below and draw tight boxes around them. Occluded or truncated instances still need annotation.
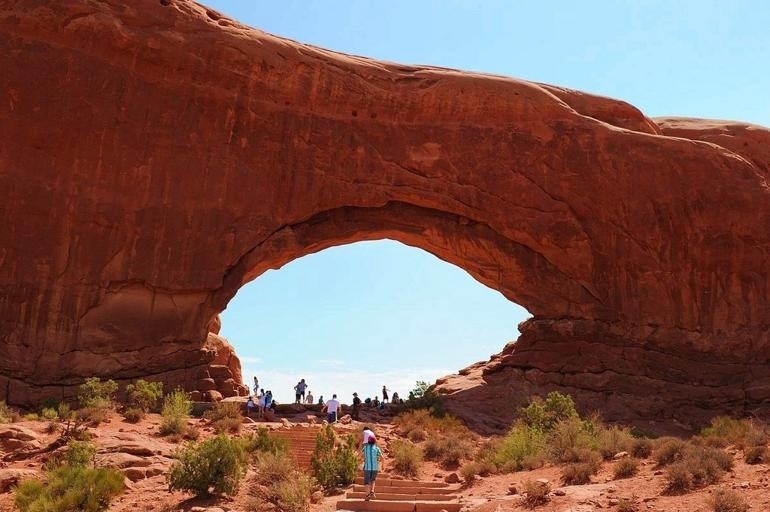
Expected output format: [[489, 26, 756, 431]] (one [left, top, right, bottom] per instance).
[[365, 492, 376, 501]]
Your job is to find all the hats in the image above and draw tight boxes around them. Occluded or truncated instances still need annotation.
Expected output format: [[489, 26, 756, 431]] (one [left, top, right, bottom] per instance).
[[368, 435, 376, 443], [352, 392, 358, 396]]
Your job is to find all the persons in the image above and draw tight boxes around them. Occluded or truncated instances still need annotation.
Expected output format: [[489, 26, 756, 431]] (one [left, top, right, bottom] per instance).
[[321, 393, 342, 423], [318, 395, 323, 405], [299, 379, 307, 404], [362, 427, 376, 446], [352, 391, 362, 421], [305, 390, 313, 403], [253, 376, 259, 391], [361, 436, 381, 501], [246, 389, 278, 416], [294, 382, 301, 403], [371, 385, 414, 422]]
